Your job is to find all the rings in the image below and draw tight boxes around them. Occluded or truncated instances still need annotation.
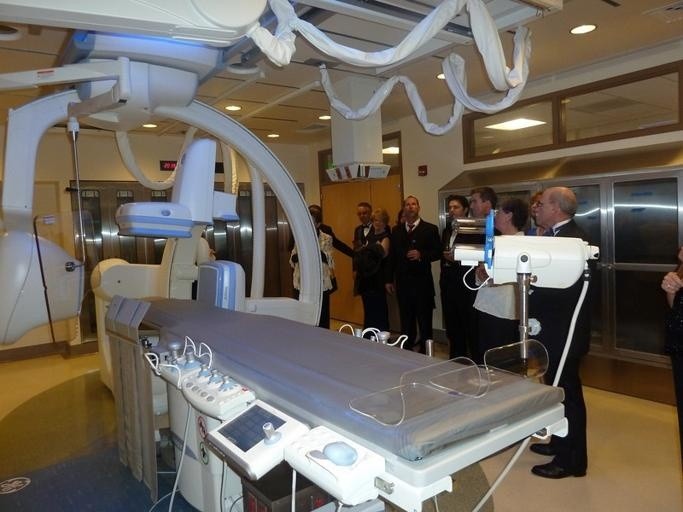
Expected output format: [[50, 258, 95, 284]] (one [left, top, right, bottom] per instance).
[[667, 284, 670, 288]]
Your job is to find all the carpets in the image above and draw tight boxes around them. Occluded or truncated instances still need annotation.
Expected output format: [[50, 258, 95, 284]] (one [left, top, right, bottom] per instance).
[[0, 445, 199, 512]]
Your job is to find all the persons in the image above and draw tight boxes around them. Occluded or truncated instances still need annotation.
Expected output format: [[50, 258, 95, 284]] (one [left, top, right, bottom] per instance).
[[660, 246, 683, 466], [441, 186, 590, 478], [288, 205, 353, 329], [352, 195, 442, 356]]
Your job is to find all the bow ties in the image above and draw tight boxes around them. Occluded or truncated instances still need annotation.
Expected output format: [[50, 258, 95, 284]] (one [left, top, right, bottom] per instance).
[[362, 225, 370, 228]]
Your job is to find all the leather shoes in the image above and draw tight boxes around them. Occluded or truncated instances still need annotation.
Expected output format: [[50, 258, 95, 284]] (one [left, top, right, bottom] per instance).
[[531, 460, 586, 478], [530, 442, 558, 455]]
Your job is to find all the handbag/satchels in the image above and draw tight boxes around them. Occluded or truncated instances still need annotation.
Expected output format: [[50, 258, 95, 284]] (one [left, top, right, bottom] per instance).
[[326, 277, 337, 295]]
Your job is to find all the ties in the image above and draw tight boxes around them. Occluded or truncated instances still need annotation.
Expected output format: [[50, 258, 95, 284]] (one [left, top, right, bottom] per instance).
[[407, 224, 415, 240]]
